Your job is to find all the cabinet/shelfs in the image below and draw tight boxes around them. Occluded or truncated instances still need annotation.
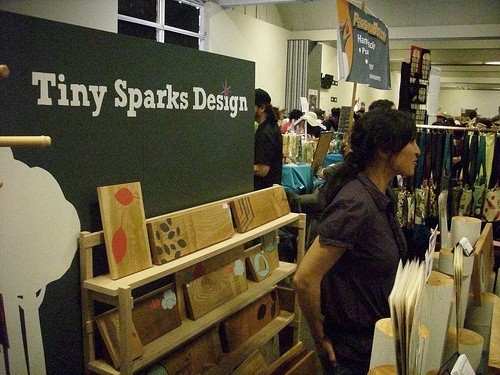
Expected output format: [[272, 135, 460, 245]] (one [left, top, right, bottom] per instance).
[[79, 184, 306, 375]]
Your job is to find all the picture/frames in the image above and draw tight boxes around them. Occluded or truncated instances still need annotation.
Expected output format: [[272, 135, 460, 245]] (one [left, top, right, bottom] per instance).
[[310, 131, 334, 170]]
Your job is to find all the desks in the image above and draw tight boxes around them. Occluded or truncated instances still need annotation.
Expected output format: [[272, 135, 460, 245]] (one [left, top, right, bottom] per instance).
[[282, 154, 343, 194]]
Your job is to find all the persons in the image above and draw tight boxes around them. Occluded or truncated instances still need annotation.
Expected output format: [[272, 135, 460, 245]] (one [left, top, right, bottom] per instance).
[[253, 89, 283, 191], [274, 98, 500, 375]]
